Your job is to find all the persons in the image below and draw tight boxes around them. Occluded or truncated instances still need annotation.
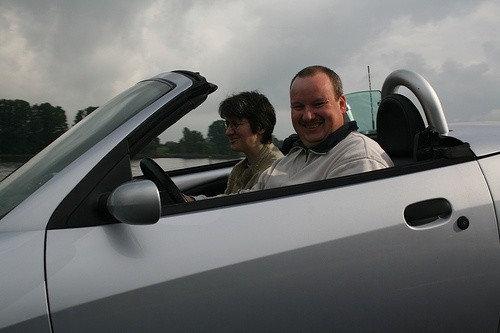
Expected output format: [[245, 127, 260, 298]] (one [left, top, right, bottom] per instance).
[[218, 90, 288, 194], [183, 65, 395, 203]]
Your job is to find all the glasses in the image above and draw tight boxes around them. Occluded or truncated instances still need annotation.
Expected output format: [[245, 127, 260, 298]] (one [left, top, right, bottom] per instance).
[[223, 120, 253, 128]]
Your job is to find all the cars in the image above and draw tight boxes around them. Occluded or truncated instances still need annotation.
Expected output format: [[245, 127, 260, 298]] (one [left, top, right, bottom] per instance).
[[0, 63, 500, 333]]
